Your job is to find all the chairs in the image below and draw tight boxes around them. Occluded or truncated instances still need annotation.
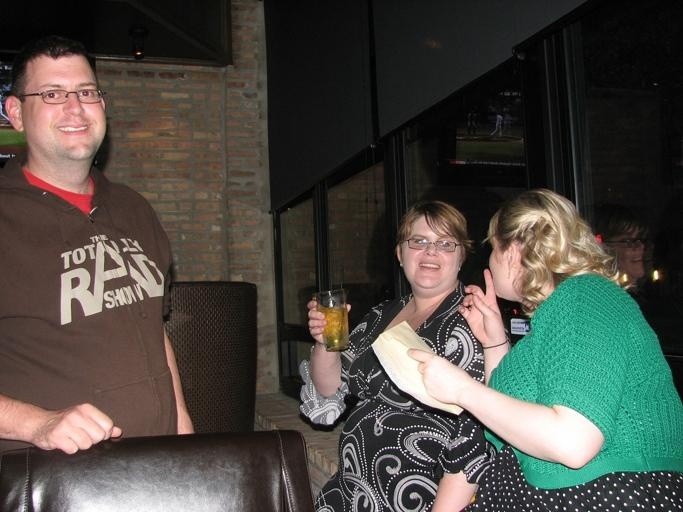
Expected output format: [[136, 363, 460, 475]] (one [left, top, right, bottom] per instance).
[[2, 428, 317, 510]]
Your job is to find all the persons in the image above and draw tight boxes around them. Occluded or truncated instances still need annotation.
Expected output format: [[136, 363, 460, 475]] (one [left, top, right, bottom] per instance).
[[1, 34, 201, 457], [465, 109, 514, 140], [582, 201, 647, 306], [404, 185, 682, 511], [299, 195, 511, 510]]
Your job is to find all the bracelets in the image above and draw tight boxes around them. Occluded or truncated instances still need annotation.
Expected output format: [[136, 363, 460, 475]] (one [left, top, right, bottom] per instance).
[[478, 339, 510, 349]]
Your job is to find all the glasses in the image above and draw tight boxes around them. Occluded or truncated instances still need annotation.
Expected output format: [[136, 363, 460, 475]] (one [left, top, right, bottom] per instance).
[[16, 87, 107, 104], [403, 238, 462, 252], [606, 239, 647, 248]]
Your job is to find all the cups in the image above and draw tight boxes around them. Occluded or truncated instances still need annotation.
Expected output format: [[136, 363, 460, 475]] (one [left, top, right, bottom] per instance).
[[311, 289, 349, 353]]
